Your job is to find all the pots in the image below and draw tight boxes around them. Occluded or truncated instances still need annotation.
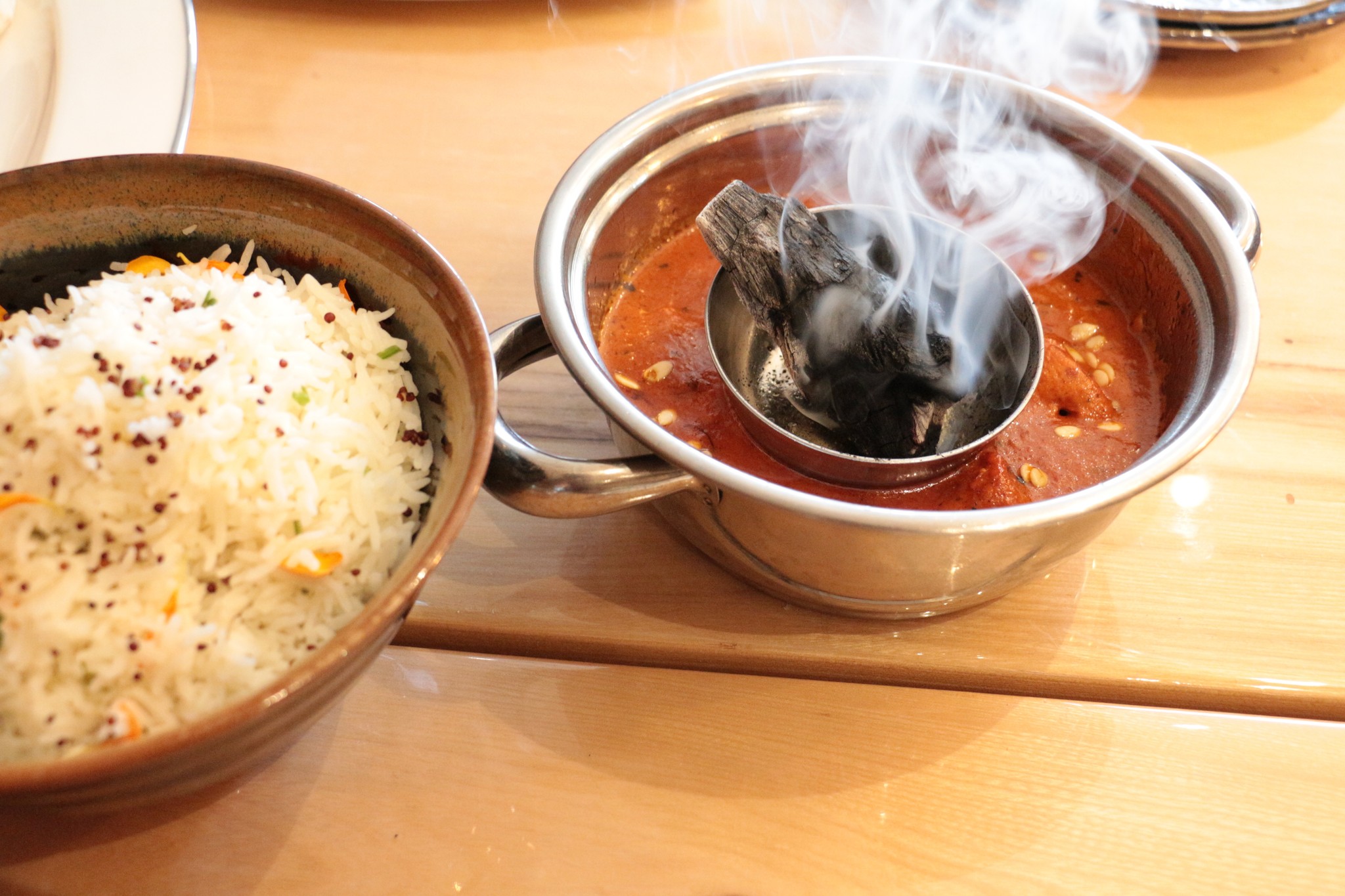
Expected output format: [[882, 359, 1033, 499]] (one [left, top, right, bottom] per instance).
[[481, 55, 1263, 620]]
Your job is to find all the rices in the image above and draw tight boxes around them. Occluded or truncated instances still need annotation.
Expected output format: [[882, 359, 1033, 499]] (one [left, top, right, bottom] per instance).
[[0, 234, 429, 776]]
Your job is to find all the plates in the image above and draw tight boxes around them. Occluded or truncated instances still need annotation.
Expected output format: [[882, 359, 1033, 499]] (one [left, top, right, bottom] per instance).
[[0, 0, 197, 174], [1119, 0, 1345, 50]]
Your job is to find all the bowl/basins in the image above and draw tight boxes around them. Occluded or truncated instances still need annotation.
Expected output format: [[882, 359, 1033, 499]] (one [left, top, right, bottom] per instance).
[[0, 156, 497, 827], [704, 203, 1044, 490]]
